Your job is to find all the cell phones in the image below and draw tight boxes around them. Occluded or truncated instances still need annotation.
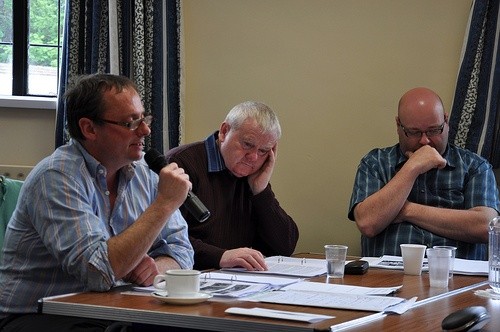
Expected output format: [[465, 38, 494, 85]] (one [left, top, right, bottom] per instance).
[[345, 261, 369, 275]]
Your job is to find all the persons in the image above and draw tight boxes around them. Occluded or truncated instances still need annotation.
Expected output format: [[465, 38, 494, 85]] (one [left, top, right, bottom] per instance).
[[0, 73, 194, 332], [161, 99, 299, 272], [347, 87, 500, 262]]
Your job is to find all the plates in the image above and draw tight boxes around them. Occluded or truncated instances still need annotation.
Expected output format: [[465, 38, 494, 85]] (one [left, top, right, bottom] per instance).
[[152, 291, 213, 305]]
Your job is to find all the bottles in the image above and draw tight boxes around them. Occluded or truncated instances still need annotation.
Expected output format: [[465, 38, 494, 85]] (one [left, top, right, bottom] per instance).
[[487, 216, 500, 294]]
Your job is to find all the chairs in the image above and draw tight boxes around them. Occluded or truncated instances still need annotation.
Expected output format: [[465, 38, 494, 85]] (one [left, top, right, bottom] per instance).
[[0, 175, 24, 252]]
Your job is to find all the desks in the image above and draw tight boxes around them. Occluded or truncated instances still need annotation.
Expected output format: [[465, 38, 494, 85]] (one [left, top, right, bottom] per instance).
[[38, 252, 500, 332]]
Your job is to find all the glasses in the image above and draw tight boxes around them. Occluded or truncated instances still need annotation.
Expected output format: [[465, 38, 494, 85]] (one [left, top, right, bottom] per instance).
[[401, 120, 446, 138], [103, 115, 152, 131]]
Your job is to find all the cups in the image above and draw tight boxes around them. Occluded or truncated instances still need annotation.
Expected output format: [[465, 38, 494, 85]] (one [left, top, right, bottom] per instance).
[[427, 287, 448, 316], [433, 246, 457, 279], [324, 244, 348, 279], [153, 269, 201, 297], [426, 248, 452, 288], [403, 275, 424, 295], [400, 244, 427, 276]]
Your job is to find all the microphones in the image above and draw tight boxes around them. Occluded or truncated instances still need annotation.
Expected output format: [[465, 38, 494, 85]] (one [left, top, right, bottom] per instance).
[[143, 148, 211, 223]]
